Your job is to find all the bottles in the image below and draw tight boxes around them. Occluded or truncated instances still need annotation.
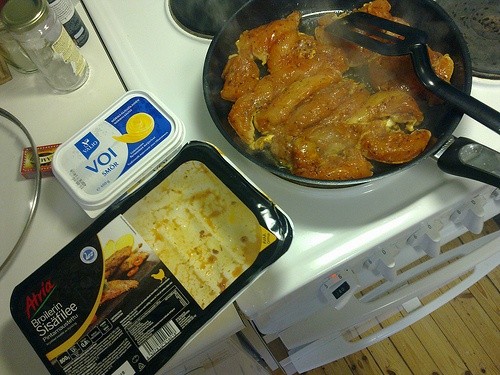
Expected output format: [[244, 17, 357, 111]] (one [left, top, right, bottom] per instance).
[[1, 0, 90, 93], [47, 0, 89, 49]]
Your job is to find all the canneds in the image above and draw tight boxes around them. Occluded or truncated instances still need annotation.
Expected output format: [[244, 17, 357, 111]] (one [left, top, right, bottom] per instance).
[[47, 0, 90, 49]]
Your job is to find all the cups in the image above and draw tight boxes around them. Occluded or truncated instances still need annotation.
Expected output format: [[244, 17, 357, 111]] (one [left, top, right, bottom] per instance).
[[0, 22, 38, 74]]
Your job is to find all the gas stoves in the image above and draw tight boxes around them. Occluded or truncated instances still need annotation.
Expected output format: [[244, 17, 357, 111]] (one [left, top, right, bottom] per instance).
[[81, 0, 499, 375]]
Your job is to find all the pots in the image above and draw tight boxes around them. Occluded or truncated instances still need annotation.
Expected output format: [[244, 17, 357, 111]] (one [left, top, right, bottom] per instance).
[[202, 0, 499, 190]]
